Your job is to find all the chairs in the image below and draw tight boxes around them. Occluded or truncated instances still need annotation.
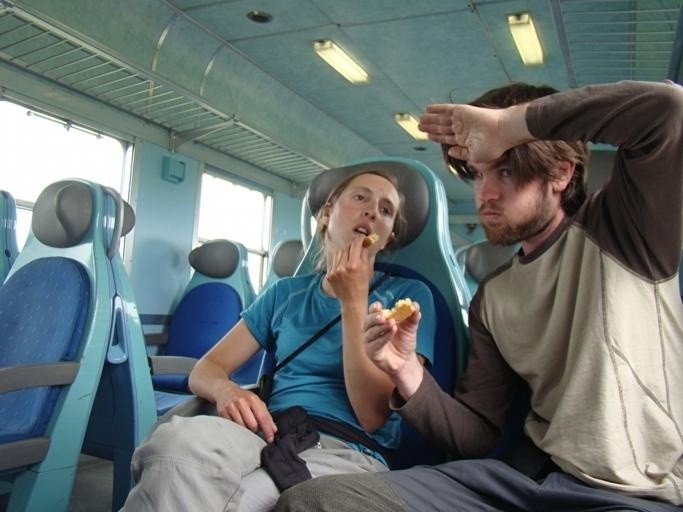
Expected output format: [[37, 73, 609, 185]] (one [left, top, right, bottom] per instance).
[[0, 143, 618, 512]]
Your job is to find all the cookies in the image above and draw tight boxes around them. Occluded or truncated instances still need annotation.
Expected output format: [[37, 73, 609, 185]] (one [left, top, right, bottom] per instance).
[[362, 233, 380, 249], [385, 296, 415, 322]]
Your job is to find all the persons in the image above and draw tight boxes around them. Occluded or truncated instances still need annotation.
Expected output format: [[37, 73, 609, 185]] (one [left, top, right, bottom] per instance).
[[117, 159, 437, 512], [276, 74, 683, 512]]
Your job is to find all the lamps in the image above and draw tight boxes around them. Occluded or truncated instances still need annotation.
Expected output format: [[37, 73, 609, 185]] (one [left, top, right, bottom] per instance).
[[506, 11, 543, 66], [311, 40, 372, 86], [395, 112, 429, 142]]
